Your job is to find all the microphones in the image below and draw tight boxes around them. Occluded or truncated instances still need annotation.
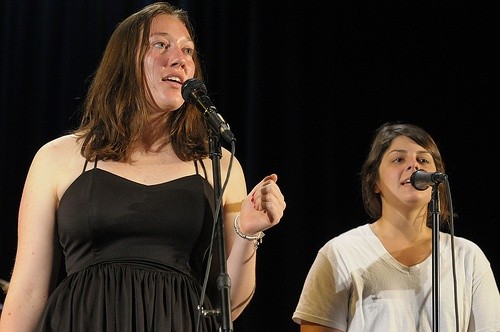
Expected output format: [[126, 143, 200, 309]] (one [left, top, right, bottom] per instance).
[[410, 169, 447, 191], [181, 78, 236, 143]]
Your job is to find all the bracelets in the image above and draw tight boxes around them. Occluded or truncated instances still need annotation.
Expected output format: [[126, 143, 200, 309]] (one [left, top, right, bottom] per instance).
[[233, 215, 265, 250]]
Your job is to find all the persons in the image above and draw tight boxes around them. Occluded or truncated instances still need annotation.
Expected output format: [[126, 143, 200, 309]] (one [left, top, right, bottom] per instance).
[[0, 2, 286, 332], [292, 122, 500, 332]]
